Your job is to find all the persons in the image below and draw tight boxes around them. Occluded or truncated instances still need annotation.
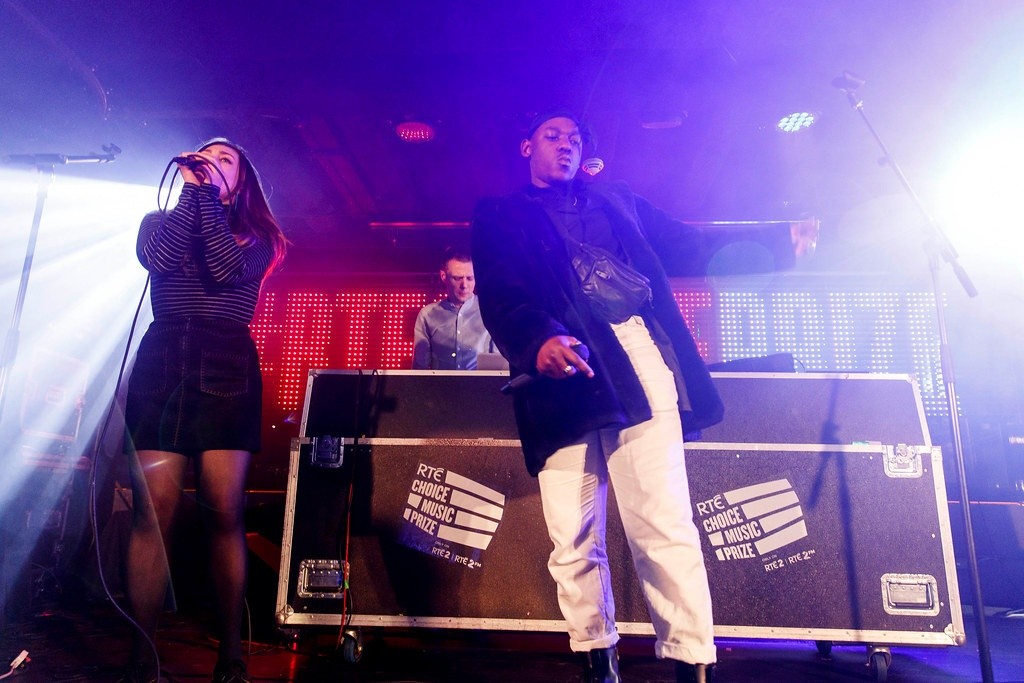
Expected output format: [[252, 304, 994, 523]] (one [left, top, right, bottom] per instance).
[[411, 245, 502, 369], [120, 134, 286, 683], [467, 106, 821, 682]]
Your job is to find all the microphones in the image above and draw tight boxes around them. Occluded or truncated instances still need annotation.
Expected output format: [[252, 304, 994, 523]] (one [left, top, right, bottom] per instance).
[[501, 344, 590, 395], [173, 156, 199, 168]]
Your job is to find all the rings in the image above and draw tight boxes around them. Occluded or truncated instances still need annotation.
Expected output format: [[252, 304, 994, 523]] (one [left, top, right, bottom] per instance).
[[562, 364, 574, 374]]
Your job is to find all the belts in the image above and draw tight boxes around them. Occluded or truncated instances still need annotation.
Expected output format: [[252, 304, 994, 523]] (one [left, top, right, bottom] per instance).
[[155, 318, 250, 331]]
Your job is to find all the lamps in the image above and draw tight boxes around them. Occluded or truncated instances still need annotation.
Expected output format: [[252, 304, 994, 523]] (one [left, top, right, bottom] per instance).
[[637, 102, 688, 131], [772, 107, 822, 135], [389, 108, 444, 142]]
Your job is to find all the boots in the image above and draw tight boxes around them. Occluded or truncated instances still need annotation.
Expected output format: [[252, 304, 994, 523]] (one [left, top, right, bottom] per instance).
[[579, 648, 623, 683], [676, 663, 716, 683]]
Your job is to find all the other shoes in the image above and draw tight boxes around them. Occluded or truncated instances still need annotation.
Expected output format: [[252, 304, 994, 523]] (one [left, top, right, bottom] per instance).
[[213, 662, 251, 683]]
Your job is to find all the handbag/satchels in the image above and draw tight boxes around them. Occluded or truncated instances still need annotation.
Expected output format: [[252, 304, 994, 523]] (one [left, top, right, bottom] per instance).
[[571, 244, 652, 324]]
